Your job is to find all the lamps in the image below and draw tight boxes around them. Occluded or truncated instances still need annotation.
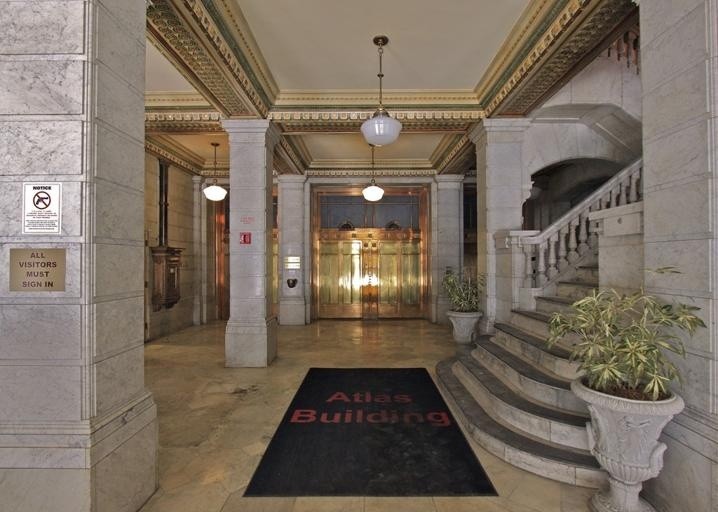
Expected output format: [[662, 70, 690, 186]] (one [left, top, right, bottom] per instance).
[[359, 35, 403, 203], [201, 141, 228, 202]]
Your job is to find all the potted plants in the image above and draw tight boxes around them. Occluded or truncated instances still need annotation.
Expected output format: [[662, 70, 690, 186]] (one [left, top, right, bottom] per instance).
[[542, 264, 706, 512]]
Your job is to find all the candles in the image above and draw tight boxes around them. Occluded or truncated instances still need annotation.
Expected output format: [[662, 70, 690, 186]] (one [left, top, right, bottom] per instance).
[[443, 265, 487, 344]]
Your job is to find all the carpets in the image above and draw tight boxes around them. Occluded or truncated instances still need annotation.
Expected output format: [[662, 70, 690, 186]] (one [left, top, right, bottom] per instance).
[[242, 368, 501, 497]]
